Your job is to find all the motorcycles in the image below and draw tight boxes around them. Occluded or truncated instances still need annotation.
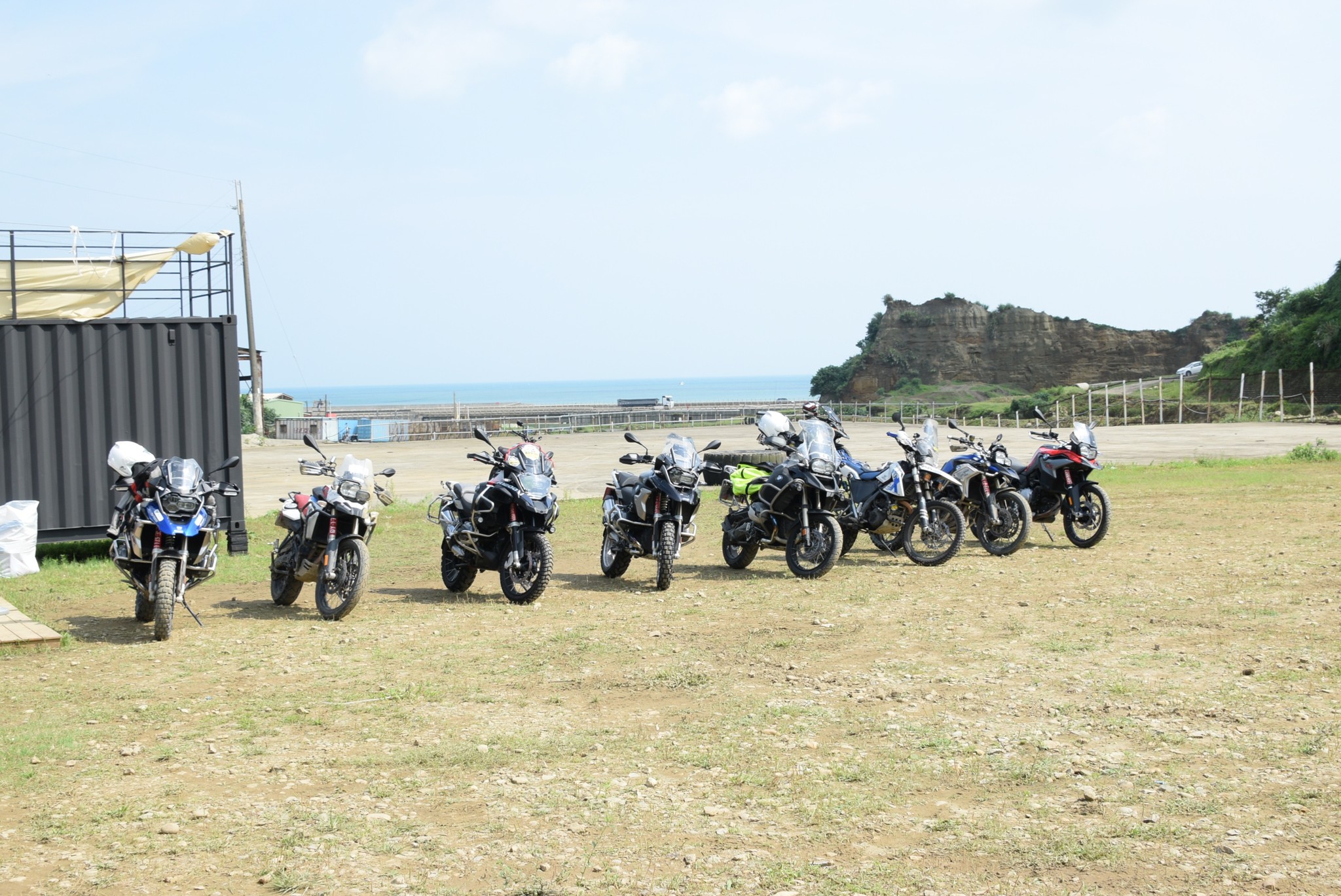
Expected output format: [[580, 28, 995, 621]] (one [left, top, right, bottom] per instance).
[[427, 419, 567, 598], [1007, 406, 1111, 549], [944, 421, 1043, 547], [597, 427, 721, 589], [714, 409, 858, 581], [828, 409, 975, 563], [107, 440, 245, 640], [266, 434, 414, 608]]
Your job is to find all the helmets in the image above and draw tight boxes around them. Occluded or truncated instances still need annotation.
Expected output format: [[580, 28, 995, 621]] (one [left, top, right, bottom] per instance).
[[107, 441, 156, 478], [803, 401, 819, 417], [758, 410, 796, 438]]
